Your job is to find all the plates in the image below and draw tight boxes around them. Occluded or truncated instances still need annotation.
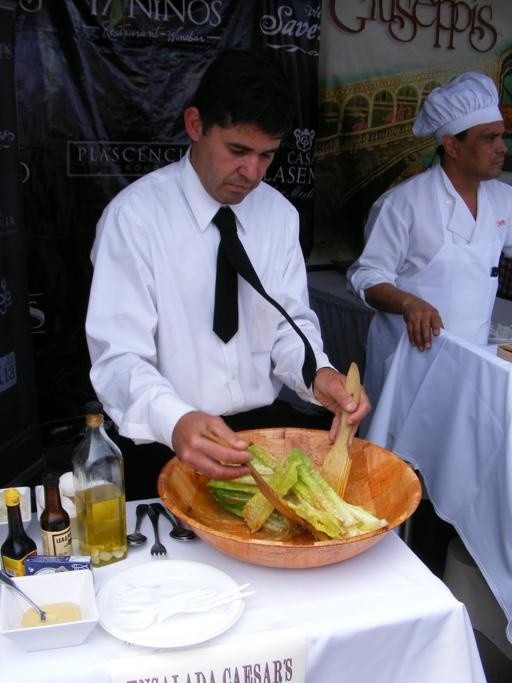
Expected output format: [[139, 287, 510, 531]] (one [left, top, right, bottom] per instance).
[[487, 335, 512, 344], [35, 470, 74, 539], [93, 560, 247, 649]]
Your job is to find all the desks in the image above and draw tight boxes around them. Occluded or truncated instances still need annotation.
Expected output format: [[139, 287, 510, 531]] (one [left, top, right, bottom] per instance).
[[1, 495, 493, 683]]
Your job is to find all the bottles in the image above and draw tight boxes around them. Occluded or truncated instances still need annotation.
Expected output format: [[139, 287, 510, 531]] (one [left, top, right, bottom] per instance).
[[0, 487, 37, 578], [38, 470, 72, 558], [71, 400, 128, 574]]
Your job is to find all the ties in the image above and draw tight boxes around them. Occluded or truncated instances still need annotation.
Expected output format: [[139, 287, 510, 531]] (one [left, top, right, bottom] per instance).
[[211, 205, 317, 390]]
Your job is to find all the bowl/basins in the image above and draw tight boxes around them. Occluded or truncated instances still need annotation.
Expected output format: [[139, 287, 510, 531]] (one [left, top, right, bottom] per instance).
[[0, 486, 32, 540], [156, 427, 425, 571], [1, 573, 94, 652]]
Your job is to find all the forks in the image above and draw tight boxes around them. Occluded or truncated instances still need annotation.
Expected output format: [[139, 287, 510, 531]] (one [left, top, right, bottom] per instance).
[[148, 503, 166, 556]]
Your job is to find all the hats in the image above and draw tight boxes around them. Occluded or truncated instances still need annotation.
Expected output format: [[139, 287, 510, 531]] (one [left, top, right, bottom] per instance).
[[411, 70, 506, 149]]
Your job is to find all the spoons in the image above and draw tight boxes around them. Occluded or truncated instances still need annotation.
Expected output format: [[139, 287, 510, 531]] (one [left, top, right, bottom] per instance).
[[124, 499, 150, 547], [116, 581, 256, 632], [156, 501, 195, 541]]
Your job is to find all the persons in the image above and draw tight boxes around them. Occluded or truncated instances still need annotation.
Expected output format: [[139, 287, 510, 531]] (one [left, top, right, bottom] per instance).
[[345, 70, 512, 568], [85, 45, 372, 500]]
